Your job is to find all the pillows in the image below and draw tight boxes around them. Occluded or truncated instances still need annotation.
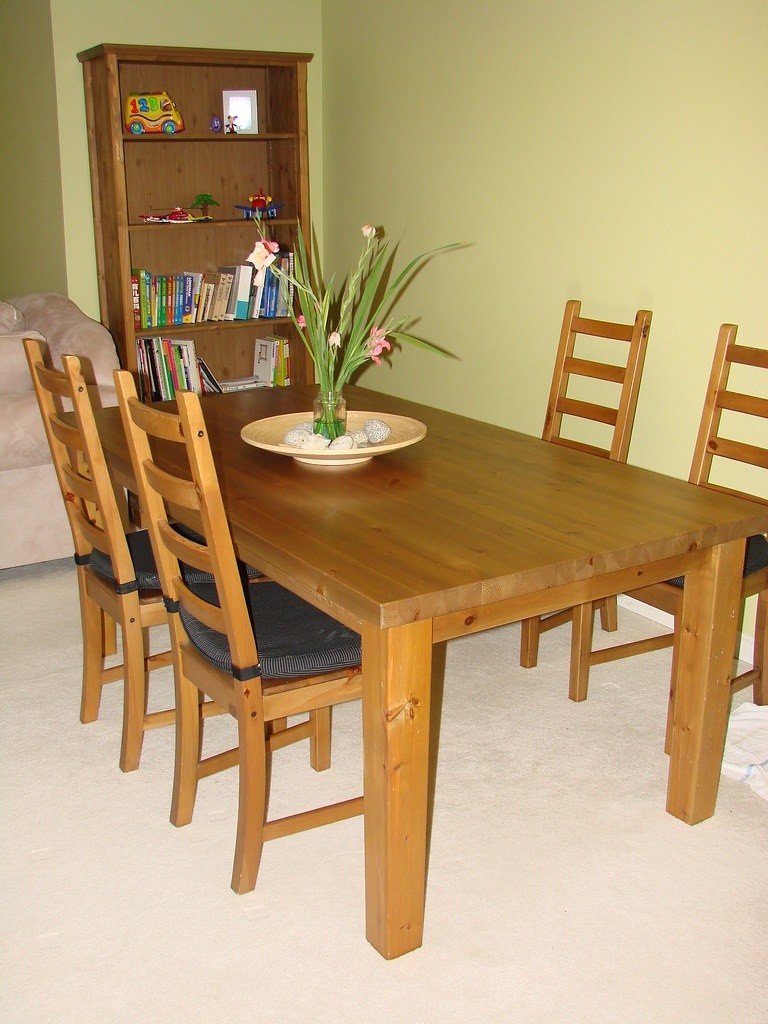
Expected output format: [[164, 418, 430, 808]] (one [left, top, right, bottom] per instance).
[[0, 330, 47, 393], [0, 300, 25, 333]]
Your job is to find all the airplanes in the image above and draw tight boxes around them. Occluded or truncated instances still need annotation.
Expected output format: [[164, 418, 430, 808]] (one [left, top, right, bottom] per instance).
[[233, 187, 284, 219], [188, 214, 214, 221]]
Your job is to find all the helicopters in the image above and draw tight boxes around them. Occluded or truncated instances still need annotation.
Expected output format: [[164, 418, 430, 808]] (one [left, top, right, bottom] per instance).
[[138, 207, 210, 224]]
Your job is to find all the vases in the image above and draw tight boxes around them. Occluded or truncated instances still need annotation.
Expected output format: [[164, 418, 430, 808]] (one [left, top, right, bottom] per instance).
[[312, 390, 347, 441]]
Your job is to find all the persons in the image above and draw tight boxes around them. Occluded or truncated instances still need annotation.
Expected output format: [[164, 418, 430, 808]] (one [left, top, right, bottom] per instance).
[[226, 116, 237, 134]]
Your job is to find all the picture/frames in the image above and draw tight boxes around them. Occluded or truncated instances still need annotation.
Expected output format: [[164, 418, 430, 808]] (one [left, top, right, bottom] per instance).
[[222, 90, 258, 135]]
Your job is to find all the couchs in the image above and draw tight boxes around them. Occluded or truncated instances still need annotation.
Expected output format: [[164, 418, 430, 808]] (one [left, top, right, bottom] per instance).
[[0, 292, 120, 571]]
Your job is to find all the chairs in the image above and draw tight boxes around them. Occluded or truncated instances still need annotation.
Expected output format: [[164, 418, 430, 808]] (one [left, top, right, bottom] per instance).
[[521, 301, 653, 668], [567, 324, 768, 757], [112, 367, 365, 894], [23, 337, 288, 773]]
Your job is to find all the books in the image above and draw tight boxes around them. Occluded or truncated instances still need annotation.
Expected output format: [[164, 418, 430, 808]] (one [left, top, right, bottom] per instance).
[[253, 335, 291, 387], [135, 337, 223, 403], [219, 376, 265, 392], [132, 249, 294, 329]]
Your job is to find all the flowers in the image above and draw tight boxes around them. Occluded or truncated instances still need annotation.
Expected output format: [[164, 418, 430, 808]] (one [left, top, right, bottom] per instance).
[[246, 206, 467, 444]]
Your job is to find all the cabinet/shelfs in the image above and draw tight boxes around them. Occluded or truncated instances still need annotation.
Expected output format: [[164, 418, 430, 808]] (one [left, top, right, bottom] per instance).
[[77, 43, 314, 402]]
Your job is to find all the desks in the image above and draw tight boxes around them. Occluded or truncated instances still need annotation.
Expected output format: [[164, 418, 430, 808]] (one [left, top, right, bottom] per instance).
[[61, 384, 768, 960]]
